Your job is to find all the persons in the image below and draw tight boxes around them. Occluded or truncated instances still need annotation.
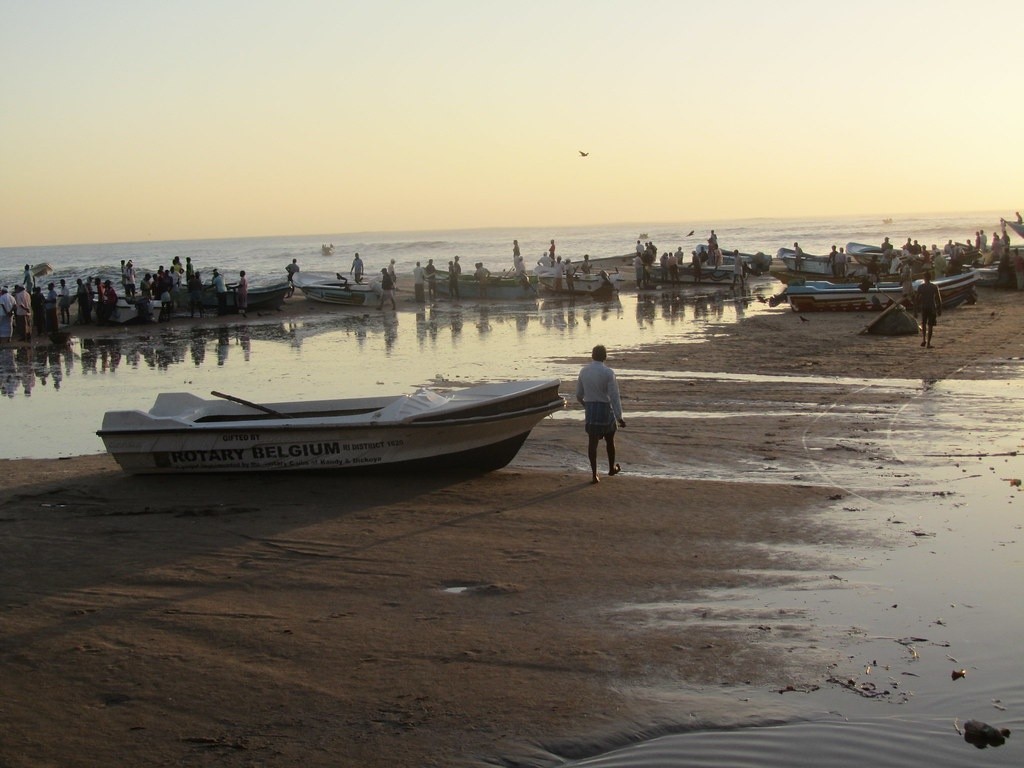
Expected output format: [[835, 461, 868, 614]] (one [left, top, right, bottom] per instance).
[[449, 256, 461, 297], [513, 240, 527, 275], [474, 263, 491, 299], [868, 219, 1024, 291], [285, 259, 299, 297], [351, 253, 364, 282], [794, 242, 803, 272], [635, 230, 723, 287], [731, 249, 744, 288], [1016, 212, 1022, 225], [576, 346, 627, 484], [0, 264, 117, 343], [414, 259, 436, 302], [827, 246, 848, 277], [376, 259, 398, 310], [917, 273, 942, 348], [537, 240, 592, 296], [123, 255, 247, 322]]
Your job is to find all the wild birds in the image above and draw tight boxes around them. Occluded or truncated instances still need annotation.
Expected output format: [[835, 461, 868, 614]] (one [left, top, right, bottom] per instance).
[[686, 230, 694, 236], [578, 151, 589, 156], [990, 312, 994, 318], [799, 316, 810, 323]]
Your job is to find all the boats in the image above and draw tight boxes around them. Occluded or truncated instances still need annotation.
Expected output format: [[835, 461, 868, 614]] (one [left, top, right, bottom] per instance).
[[54, 264, 388, 323], [94, 378, 568, 485], [416, 243, 773, 299], [778, 216, 1024, 313]]
